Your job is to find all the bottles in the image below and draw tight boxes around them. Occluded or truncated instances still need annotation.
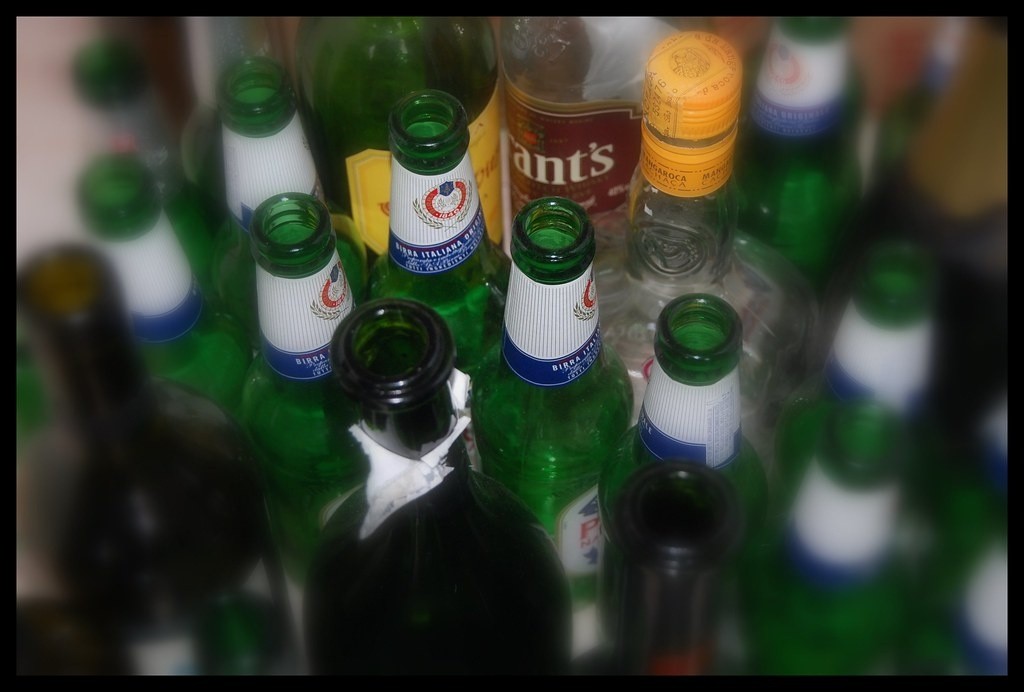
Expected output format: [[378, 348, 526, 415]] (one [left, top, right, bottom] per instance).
[[16, 13, 1006, 675]]
